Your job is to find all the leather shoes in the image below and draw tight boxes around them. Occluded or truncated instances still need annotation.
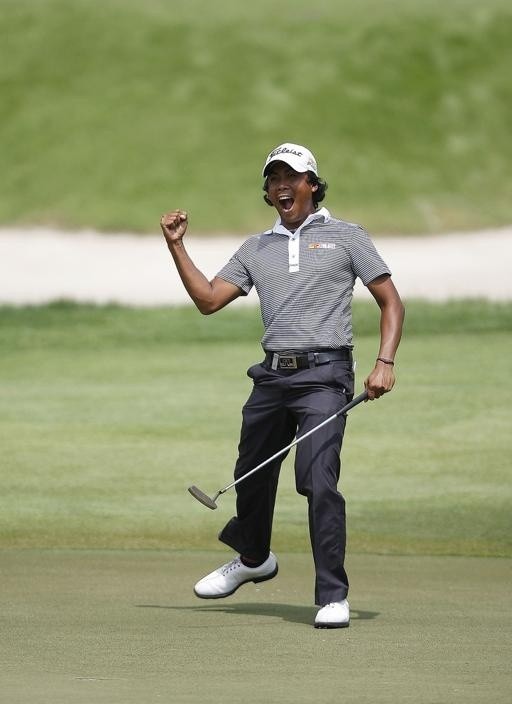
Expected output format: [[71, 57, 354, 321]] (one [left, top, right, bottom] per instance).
[[314, 599, 350, 629], [194, 551, 278, 599]]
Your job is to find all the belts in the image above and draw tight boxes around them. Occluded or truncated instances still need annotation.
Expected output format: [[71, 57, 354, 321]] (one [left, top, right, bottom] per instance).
[[264, 351, 353, 371]]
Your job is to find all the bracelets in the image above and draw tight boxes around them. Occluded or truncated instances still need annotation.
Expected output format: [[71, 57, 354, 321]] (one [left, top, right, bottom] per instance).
[[377, 357, 394, 366]]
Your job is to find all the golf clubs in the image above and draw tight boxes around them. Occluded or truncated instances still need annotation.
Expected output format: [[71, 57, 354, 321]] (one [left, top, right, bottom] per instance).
[[188, 389, 368, 510]]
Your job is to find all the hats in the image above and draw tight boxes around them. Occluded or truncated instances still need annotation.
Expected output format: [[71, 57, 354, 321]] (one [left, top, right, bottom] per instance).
[[262, 143, 318, 177]]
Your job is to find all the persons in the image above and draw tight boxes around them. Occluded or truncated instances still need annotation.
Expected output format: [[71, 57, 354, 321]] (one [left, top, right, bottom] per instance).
[[160, 142, 405, 629]]
